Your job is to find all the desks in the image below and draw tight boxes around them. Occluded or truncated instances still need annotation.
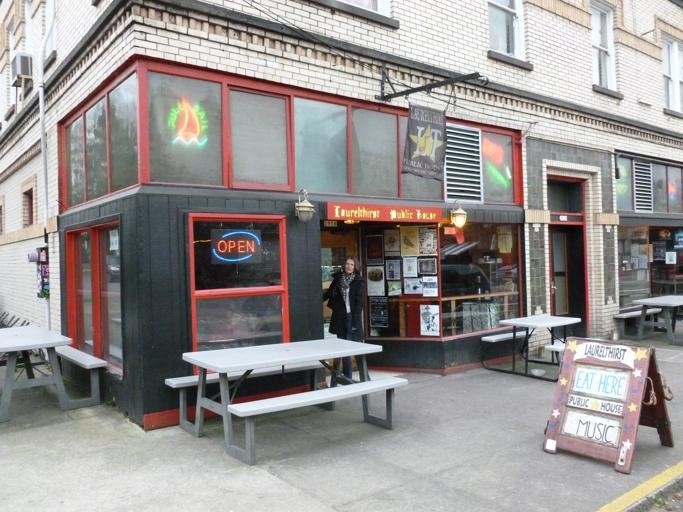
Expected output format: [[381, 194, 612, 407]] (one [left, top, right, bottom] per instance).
[[181, 338, 383, 447], [499, 314, 581, 376], [1, 325, 73, 425], [632, 294, 683, 345]]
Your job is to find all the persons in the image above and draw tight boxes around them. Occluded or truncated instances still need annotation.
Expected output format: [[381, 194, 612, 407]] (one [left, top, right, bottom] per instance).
[[242, 281, 282, 335], [322, 254, 372, 383]]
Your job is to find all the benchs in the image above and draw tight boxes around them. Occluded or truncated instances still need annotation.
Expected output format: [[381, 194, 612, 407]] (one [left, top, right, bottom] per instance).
[[612, 308, 662, 340], [164, 361, 332, 437], [228, 375, 409, 466], [536, 336, 574, 381], [55, 345, 109, 409], [480, 330, 535, 377]]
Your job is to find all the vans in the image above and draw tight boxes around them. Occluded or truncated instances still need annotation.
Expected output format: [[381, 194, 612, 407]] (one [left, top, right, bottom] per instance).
[[438, 263, 494, 328]]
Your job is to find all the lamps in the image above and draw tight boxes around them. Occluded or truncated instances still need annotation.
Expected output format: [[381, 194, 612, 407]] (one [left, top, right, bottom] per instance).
[[295, 188, 316, 224], [450, 200, 468, 228]]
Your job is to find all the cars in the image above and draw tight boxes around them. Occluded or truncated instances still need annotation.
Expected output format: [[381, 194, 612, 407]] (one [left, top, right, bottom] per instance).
[[491, 262, 518, 282], [105, 253, 122, 283]]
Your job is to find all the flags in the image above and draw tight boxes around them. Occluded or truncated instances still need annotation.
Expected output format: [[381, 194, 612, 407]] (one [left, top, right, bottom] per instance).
[[401, 104, 448, 181]]
